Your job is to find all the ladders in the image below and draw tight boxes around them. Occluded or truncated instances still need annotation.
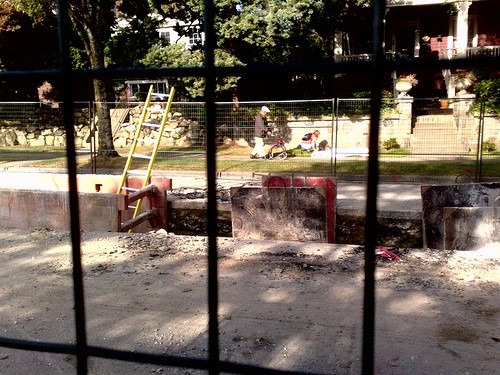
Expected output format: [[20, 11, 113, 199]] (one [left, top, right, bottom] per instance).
[[117, 85, 175, 233]]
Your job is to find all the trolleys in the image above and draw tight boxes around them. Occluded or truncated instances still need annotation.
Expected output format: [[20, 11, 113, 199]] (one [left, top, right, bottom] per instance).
[[265, 131, 288, 160]]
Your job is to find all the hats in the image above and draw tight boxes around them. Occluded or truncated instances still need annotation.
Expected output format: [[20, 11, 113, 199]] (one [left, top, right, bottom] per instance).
[[262, 106, 270, 113]]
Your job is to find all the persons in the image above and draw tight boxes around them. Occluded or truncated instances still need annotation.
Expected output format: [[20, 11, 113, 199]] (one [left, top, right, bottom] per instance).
[[301, 130, 320, 153], [251, 106, 273, 160]]
